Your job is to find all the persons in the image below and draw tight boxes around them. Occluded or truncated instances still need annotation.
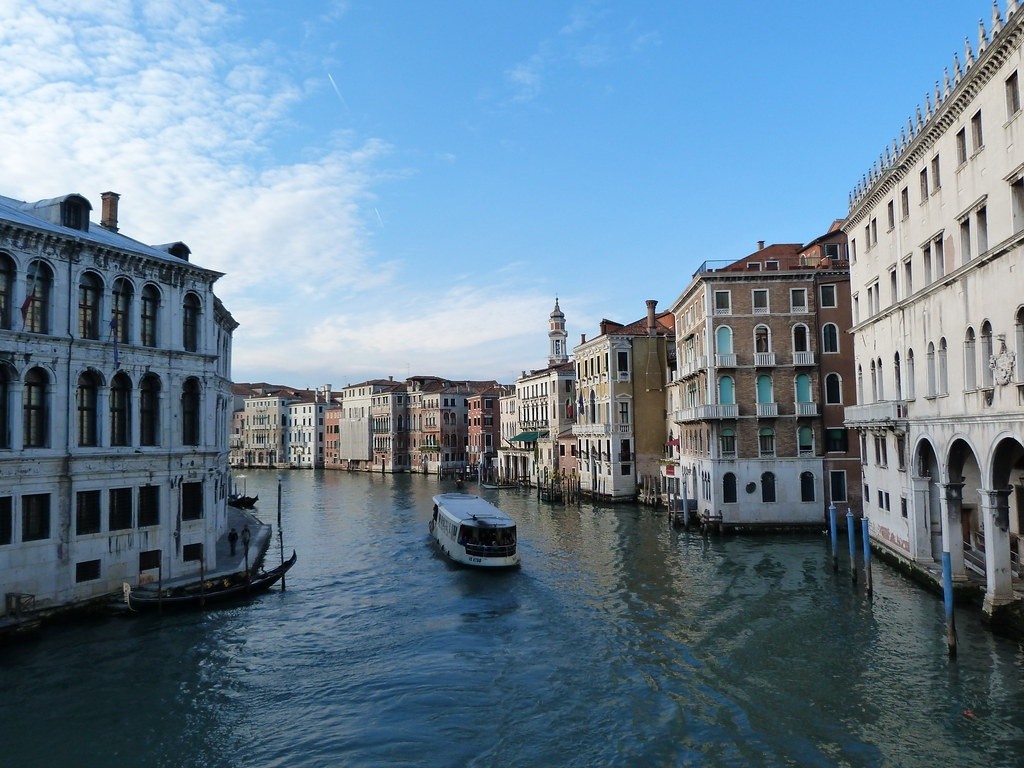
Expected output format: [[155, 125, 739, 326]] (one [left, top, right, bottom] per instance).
[[240, 524, 250, 556], [227, 527, 239, 556]]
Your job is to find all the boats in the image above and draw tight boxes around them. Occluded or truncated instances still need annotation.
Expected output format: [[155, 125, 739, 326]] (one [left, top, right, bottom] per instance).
[[429, 492, 522, 570], [122, 549, 298, 614], [227, 493, 259, 508]]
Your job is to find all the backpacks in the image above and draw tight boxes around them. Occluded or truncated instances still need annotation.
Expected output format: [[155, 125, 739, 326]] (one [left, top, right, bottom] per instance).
[[242, 529, 250, 544]]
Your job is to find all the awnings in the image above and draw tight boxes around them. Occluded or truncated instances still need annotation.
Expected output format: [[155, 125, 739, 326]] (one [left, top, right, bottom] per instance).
[[509, 430, 549, 442], [665, 438, 679, 446]]
